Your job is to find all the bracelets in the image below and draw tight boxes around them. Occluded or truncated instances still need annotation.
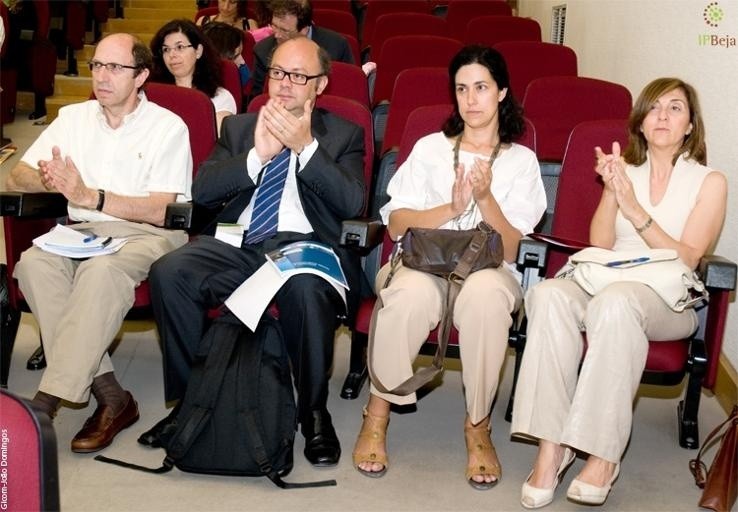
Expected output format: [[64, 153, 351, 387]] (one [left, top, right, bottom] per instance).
[[637, 219, 652, 233]]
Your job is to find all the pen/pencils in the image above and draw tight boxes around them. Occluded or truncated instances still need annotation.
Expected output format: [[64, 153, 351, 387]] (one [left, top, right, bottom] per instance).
[[607, 258, 650, 267], [83, 234, 97, 242]]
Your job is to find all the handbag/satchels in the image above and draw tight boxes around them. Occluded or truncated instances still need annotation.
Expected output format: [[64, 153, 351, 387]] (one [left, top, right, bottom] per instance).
[[567, 247, 711, 313]]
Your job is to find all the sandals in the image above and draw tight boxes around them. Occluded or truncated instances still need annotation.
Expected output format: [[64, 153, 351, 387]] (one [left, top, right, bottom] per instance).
[[351, 402, 388, 477], [465, 426, 500, 488]]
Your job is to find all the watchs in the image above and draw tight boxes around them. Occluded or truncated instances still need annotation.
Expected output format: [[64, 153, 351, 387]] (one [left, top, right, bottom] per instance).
[[96, 190, 104, 211]]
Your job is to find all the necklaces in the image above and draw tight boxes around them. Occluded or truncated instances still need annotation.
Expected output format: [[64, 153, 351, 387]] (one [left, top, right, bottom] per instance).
[[453, 131, 502, 230]]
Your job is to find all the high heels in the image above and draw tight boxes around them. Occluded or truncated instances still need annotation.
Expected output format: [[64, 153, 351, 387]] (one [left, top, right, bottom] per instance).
[[567, 451, 621, 505], [518, 443, 576, 508]]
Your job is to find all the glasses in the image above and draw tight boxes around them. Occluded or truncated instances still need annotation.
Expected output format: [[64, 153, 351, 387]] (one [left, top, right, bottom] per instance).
[[86, 60, 143, 72], [162, 44, 195, 53], [263, 65, 325, 86]]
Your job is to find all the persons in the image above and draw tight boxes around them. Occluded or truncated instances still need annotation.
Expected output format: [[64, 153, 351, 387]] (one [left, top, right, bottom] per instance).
[[4, 1, 37, 66], [5, 33, 194, 454], [509, 78, 728, 509], [201, 21, 253, 89], [135, 36, 364, 466], [144, 18, 236, 139], [355, 46, 547, 491], [195, 0, 260, 32], [252, 0, 353, 98]]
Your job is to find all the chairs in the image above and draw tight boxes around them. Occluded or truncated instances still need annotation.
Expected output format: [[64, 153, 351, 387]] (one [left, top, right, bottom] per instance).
[[452, 18, 541, 51], [330, 31, 362, 67], [1, 82, 219, 311], [208, 93, 374, 313], [240, 29, 258, 93], [506, 120, 737, 448], [306, 10, 358, 45], [297, 0, 354, 14], [63, 0, 85, 75], [518, 78, 633, 234], [374, 66, 454, 215], [93, 0, 106, 43], [0, 0, 14, 149], [338, 103, 536, 400], [360, 0, 437, 24], [264, 62, 369, 108], [27, 0, 58, 121], [436, 0, 516, 21], [0, 387, 60, 508], [203, 58, 243, 115], [491, 38, 576, 99], [194, 6, 256, 25], [363, 12, 450, 56], [369, 34, 463, 106]]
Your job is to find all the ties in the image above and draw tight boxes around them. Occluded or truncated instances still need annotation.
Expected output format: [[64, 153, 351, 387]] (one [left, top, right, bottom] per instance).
[[244, 145, 291, 244]]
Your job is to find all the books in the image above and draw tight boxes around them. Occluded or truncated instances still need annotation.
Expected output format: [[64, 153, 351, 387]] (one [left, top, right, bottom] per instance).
[[215, 222, 246, 252], [32, 224, 127, 260], [45, 233, 113, 253], [264, 242, 350, 290]]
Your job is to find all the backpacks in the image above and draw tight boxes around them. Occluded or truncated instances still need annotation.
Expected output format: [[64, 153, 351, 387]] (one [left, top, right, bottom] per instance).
[[94, 313, 338, 490]]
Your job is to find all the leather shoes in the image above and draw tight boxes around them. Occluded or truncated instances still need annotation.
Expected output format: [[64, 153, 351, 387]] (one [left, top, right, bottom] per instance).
[[136, 398, 182, 450], [70, 391, 139, 454], [301, 397, 341, 465], [25, 344, 46, 371]]
